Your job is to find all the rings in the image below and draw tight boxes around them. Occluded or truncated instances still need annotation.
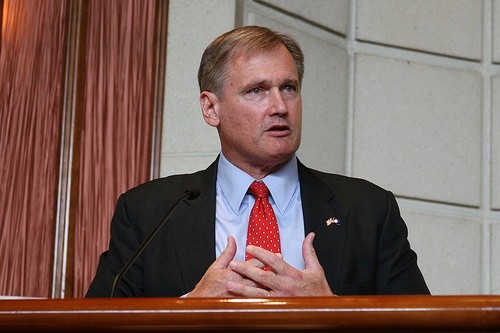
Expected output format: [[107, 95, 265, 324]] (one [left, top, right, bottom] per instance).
[[267, 291, 271, 297]]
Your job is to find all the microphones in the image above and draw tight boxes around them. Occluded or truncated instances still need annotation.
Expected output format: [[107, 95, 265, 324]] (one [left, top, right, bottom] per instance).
[[111, 186, 202, 298]]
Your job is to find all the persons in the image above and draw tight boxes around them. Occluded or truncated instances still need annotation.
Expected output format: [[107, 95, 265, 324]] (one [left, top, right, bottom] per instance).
[[81, 25, 432, 300]]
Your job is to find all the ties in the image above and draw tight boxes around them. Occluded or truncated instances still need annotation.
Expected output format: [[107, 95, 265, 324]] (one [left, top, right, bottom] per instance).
[[246, 180, 281, 292]]
